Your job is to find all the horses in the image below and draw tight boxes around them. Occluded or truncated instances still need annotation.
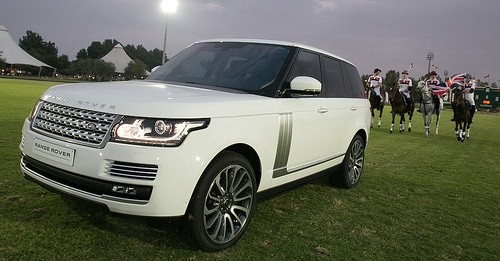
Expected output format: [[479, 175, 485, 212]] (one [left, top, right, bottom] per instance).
[[362, 80, 386, 128], [387, 80, 415, 134], [453, 88, 473, 142], [415, 77, 444, 136]]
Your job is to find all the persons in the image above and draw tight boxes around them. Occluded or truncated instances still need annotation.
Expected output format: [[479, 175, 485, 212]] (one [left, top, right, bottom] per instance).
[[365, 68, 477, 123]]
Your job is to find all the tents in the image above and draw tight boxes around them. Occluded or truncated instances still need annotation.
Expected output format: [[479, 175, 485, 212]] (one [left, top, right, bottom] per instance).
[[101, 42, 152, 75], [0, 24, 57, 79]]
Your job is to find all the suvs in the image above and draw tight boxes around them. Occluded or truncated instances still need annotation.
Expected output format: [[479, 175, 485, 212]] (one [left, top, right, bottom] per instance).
[[19, 39, 372, 254]]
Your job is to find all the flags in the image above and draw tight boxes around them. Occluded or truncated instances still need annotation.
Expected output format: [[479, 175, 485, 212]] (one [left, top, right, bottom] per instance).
[[429, 71, 466, 95]]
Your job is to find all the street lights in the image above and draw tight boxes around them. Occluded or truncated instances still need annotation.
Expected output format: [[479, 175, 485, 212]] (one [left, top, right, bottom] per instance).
[[159, 0, 180, 67]]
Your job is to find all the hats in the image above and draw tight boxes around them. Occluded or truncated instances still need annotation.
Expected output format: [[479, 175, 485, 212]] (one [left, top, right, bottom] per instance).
[[430, 71, 437, 75], [374, 68, 381, 73], [402, 70, 409, 75]]
